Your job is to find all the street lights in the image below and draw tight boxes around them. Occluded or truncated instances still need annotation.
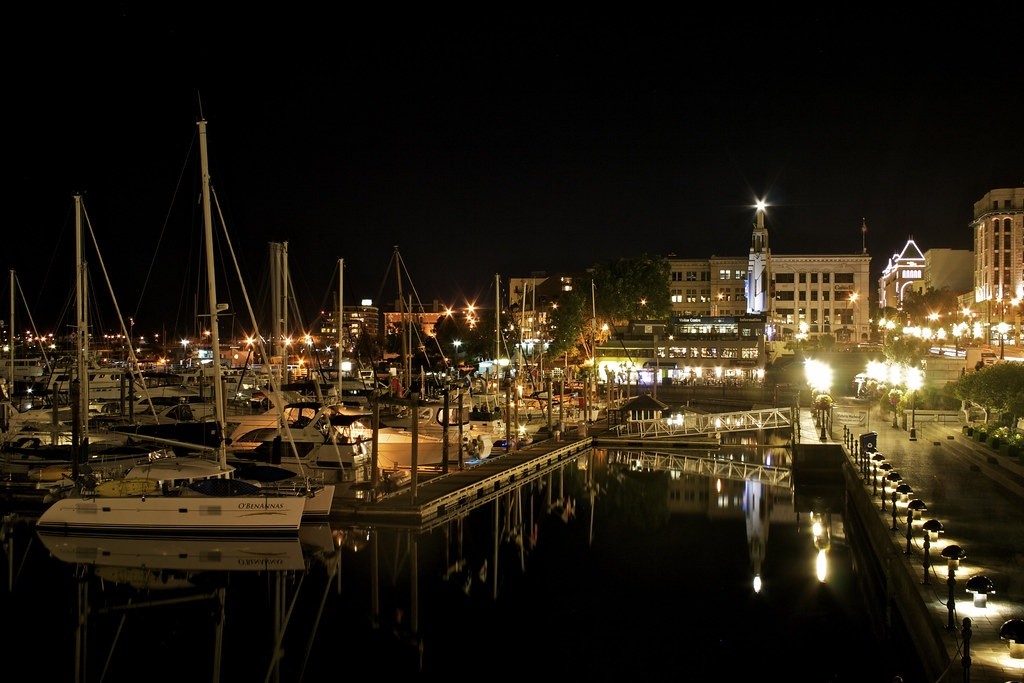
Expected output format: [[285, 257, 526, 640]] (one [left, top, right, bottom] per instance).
[[862, 357, 925, 442], [801, 356, 838, 441]]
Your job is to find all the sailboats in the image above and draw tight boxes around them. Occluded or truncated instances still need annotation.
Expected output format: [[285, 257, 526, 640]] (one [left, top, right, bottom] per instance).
[[39, 533, 342, 683], [0, 119, 474, 528]]
